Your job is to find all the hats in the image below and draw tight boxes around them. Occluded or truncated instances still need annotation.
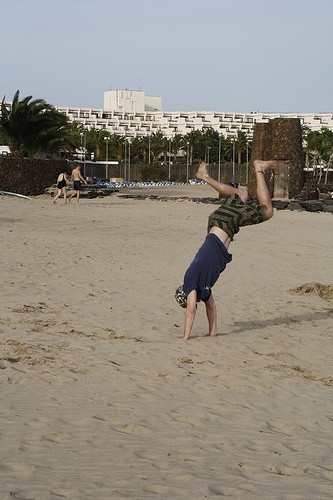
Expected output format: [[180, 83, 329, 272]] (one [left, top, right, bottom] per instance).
[[174, 284, 187, 309]]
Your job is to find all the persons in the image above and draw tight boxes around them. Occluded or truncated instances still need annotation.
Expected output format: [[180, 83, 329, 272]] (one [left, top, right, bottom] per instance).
[[173, 159, 279, 340], [68, 164, 87, 205], [53, 168, 71, 204]]
